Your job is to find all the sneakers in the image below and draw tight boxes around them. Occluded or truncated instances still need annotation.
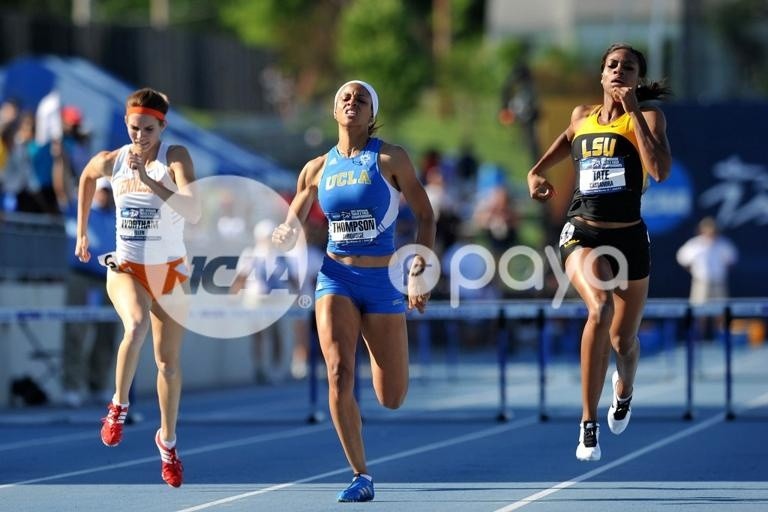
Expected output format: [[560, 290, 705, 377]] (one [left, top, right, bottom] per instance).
[[575, 420, 601, 461], [607, 370, 633, 435], [154, 429, 183, 487], [102, 394, 130, 448], [339, 471, 374, 502]]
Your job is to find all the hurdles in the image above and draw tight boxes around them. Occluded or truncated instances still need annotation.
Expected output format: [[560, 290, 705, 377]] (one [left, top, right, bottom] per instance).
[[1, 298, 764, 423]]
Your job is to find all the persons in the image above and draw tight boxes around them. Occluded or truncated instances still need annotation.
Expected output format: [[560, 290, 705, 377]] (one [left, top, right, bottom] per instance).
[[675, 211, 740, 332], [273, 79, 437, 501], [526, 43, 673, 463], [76, 89, 200, 487], [3, 91, 516, 295]]
